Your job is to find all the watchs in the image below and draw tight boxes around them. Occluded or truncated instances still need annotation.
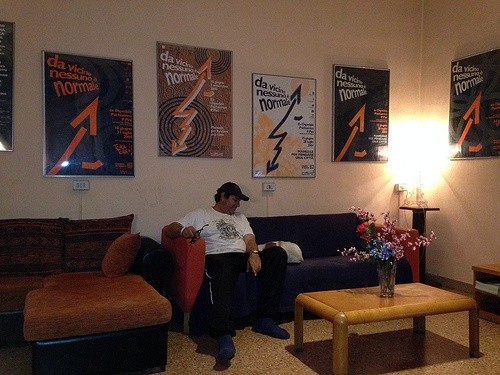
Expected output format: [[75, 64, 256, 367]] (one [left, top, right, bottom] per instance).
[[250, 249, 258, 255]]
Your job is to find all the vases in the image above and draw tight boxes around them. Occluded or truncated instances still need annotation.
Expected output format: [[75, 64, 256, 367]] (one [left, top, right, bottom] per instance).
[[376, 260, 396, 298]]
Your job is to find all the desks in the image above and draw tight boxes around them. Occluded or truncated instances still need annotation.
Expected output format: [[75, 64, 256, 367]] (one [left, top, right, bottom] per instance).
[[400, 206, 440, 284]]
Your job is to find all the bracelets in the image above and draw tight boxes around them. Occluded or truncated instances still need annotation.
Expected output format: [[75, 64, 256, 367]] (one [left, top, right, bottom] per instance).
[[180, 226, 186, 237]]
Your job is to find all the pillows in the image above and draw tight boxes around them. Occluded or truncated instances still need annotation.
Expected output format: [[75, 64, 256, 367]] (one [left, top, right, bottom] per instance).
[[0, 218, 69, 276], [101, 231, 141, 278], [58, 214, 134, 272]]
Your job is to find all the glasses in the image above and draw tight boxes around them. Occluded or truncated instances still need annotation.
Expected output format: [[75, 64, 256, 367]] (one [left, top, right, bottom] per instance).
[[189, 224, 209, 244]]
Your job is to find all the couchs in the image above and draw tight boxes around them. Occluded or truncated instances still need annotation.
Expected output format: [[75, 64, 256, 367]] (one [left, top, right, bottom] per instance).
[[162, 213, 420, 334], [0, 236, 174, 375]]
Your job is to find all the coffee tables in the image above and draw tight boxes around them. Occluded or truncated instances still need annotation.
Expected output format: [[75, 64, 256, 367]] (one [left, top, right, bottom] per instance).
[[294, 283, 479, 375]]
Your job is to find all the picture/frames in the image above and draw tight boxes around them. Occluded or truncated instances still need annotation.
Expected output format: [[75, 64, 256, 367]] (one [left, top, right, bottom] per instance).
[[0, 20, 15, 152], [251, 73, 317, 179], [41, 49, 135, 178], [448, 47, 500, 161], [155, 41, 234, 158], [332, 63, 391, 164]]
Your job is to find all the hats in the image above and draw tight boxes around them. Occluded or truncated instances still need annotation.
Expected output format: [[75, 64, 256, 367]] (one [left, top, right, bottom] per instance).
[[218, 181, 249, 201]]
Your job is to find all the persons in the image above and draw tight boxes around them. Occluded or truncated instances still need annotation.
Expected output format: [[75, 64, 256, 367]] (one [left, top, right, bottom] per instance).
[[164, 182, 288, 360]]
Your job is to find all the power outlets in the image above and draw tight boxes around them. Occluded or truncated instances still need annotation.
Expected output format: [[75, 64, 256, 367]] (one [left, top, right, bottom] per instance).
[[72, 180, 90, 191], [263, 183, 277, 191], [397, 183, 409, 192]]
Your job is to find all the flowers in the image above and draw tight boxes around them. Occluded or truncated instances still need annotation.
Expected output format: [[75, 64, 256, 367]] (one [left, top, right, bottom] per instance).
[[337, 206, 437, 290]]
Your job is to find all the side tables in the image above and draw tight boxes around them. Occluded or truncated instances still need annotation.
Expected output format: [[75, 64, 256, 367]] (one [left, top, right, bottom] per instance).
[[472, 265, 500, 323]]
